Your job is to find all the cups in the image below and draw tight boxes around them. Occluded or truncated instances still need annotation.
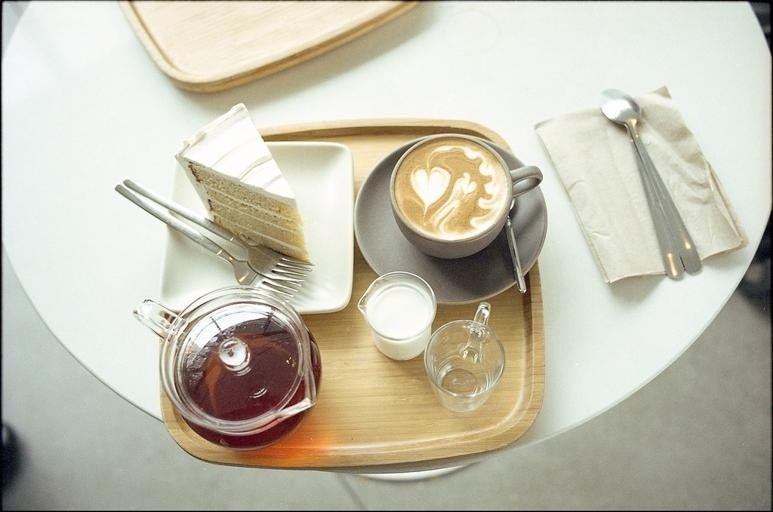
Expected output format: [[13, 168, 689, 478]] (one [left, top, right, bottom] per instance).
[[423, 301, 509, 418], [388, 132, 542, 259], [356, 269, 438, 363]]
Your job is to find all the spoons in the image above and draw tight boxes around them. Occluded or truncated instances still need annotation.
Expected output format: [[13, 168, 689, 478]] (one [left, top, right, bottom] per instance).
[[602, 93, 686, 282], [600, 89, 704, 276]]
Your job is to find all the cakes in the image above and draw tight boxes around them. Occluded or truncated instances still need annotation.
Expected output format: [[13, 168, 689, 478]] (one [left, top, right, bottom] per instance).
[[173, 103, 312, 263]]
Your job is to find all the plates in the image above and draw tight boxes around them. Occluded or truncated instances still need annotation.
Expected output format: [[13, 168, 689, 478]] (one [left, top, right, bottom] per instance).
[[354, 132, 550, 308], [160, 140, 356, 315]]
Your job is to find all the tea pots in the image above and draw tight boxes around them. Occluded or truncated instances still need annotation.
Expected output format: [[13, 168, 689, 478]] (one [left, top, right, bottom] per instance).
[[131, 287, 321, 450]]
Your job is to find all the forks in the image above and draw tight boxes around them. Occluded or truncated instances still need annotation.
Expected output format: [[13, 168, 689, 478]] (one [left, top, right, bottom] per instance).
[[123, 177, 315, 285], [113, 185, 309, 300]]
